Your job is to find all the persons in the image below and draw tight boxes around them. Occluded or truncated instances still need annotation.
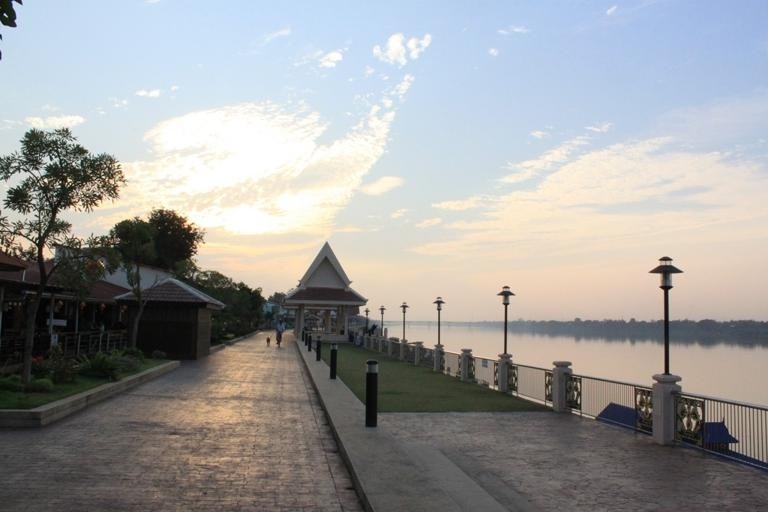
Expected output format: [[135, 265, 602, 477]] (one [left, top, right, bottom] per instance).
[[276, 319, 285, 344]]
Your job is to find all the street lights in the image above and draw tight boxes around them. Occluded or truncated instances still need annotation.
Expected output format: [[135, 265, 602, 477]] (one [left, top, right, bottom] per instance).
[[398, 299, 410, 339], [431, 294, 447, 345], [378, 304, 388, 334], [495, 283, 517, 355], [363, 306, 371, 332], [648, 254, 685, 378]]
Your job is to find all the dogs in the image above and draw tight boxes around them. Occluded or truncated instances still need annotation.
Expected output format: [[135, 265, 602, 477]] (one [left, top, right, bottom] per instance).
[[266, 336, 270, 345]]
